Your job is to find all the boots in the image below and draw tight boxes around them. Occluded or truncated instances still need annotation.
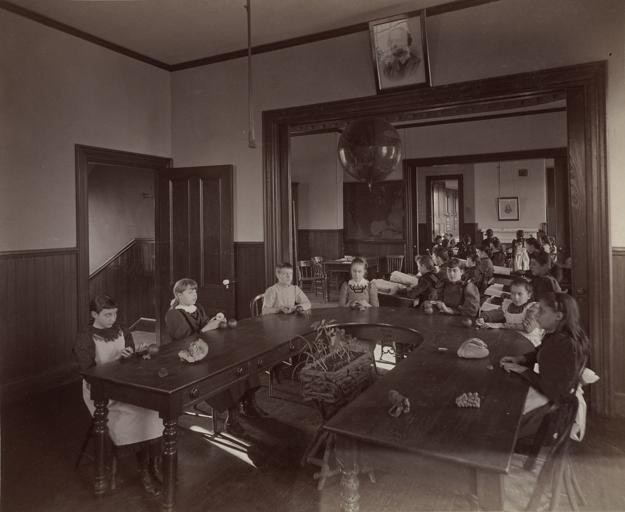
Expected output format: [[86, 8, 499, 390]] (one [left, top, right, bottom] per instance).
[[133, 456, 164, 497]]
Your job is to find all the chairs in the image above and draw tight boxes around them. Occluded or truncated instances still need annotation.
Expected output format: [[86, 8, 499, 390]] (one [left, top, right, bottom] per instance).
[[75, 417, 162, 489], [338, 281, 378, 374], [385, 255, 405, 281], [312, 256, 336, 289], [193, 403, 227, 434], [297, 260, 326, 300], [515, 356, 589, 505], [467, 396, 579, 511], [250, 294, 281, 397]]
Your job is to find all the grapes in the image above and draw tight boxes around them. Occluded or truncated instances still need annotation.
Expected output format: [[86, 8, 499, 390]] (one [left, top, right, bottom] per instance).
[[454, 391, 481, 409]]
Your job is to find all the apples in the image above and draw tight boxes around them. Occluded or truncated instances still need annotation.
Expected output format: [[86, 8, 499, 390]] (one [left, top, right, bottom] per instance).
[[228, 319, 238, 328], [462, 317, 472, 328], [148, 343, 159, 355], [296, 306, 304, 313], [423, 307, 433, 315], [349, 301, 358, 309], [219, 322, 227, 329]]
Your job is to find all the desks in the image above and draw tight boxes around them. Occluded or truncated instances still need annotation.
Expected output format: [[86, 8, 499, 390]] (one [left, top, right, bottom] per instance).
[[325, 255, 380, 302], [380, 241, 571, 332], [80, 306, 538, 512], [349, 239, 404, 257]]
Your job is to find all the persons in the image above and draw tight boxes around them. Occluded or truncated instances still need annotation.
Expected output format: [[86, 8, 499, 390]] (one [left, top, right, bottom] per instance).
[[380, 27, 421, 82], [260, 262, 313, 377], [337, 256, 380, 349], [76, 292, 167, 499], [407, 228, 589, 447], [163, 278, 270, 438]]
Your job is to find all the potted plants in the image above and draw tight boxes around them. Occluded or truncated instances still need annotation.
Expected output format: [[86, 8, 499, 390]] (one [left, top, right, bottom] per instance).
[[288, 318, 380, 404]]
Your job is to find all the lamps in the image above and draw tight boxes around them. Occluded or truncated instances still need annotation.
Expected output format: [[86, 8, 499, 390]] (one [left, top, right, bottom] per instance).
[[336, 114, 402, 189]]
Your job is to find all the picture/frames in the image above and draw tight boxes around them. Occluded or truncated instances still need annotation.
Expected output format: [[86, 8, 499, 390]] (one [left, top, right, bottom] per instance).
[[497, 197, 519, 221], [368, 8, 431, 94]]
